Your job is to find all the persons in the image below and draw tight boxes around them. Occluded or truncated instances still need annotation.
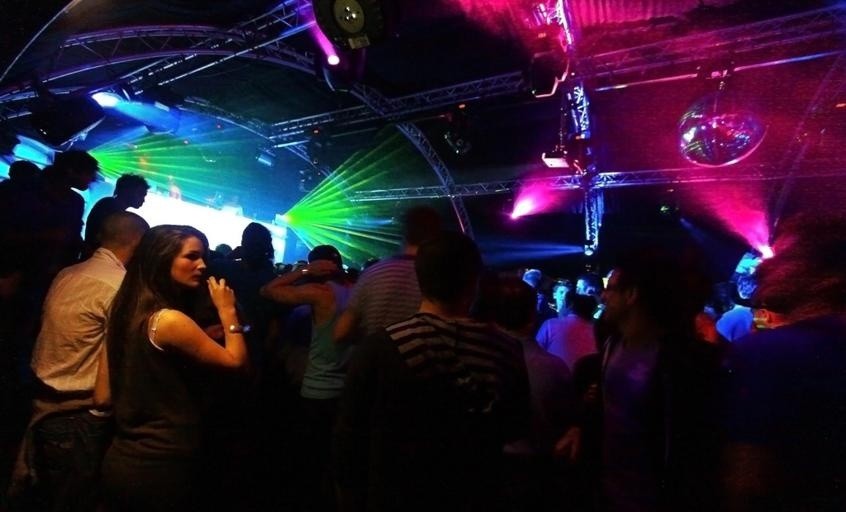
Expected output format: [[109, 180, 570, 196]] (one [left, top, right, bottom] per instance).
[[0, 146, 846, 511]]
